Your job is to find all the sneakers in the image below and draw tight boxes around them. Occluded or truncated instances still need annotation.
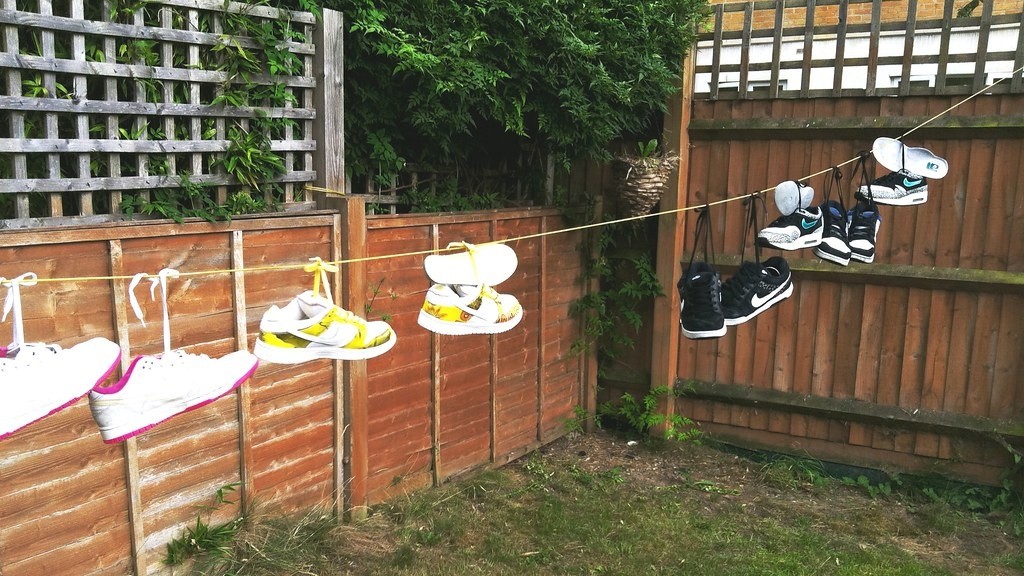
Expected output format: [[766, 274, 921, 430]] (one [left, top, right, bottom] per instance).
[[676, 262, 727, 338], [89, 349, 259, 444], [855, 169, 928, 205], [813, 201, 852, 266], [758, 205, 824, 251], [720, 256, 794, 325], [846, 201, 882, 263], [418, 283, 523, 335], [0, 336, 121, 441], [254, 290, 396, 365]]
